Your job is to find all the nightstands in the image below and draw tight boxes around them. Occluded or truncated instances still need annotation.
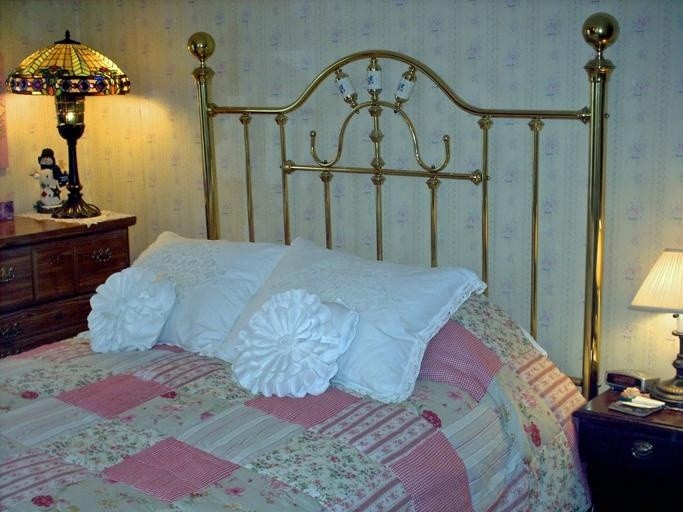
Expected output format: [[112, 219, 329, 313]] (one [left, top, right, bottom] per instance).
[[571, 387, 683, 512]]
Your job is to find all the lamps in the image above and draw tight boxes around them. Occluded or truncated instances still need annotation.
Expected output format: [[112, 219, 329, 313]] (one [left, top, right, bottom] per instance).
[[629, 249, 683, 406], [6, 28, 132, 218]]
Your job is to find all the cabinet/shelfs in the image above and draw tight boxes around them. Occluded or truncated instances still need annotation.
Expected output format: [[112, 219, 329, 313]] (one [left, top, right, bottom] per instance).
[[0, 210, 136, 361]]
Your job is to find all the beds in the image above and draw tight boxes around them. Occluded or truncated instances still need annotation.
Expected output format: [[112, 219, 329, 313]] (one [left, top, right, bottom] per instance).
[[0, 11, 621, 512]]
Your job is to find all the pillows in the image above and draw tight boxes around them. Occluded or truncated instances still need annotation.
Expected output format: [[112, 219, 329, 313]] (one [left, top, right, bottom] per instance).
[[131, 229, 290, 359], [87, 266, 176, 354], [229, 289, 359, 399], [217, 236, 478, 403]]
